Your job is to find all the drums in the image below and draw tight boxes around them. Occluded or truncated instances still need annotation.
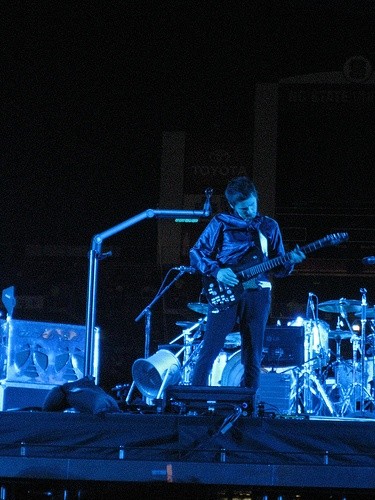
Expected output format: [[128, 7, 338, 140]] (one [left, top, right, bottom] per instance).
[[335, 361, 368, 401], [207, 350, 233, 386], [290, 319, 330, 367]]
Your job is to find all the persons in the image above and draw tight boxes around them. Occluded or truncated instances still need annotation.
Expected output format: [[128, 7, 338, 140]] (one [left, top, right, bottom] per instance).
[[190, 177, 305, 390]]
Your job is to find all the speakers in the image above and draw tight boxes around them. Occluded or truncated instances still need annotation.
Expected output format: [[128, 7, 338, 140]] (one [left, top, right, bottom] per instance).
[[165, 384, 257, 417]]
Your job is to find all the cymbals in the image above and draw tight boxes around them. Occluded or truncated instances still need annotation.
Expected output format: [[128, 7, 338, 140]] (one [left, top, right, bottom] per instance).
[[354, 308, 375, 318], [224, 332, 241, 341], [187, 302, 208, 315], [175, 321, 202, 330], [318, 299, 370, 313], [328, 330, 358, 340]]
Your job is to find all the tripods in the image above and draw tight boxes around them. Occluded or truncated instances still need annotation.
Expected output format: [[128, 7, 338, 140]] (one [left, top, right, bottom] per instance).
[[124, 271, 184, 403], [292, 293, 375, 419]]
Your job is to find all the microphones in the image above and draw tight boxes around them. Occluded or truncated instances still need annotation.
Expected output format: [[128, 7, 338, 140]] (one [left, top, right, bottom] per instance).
[[203, 186, 213, 214], [174, 266, 196, 274], [306, 293, 313, 317]]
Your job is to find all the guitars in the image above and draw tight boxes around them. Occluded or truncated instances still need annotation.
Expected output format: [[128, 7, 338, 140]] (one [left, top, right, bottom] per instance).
[[203, 232, 349, 311]]
